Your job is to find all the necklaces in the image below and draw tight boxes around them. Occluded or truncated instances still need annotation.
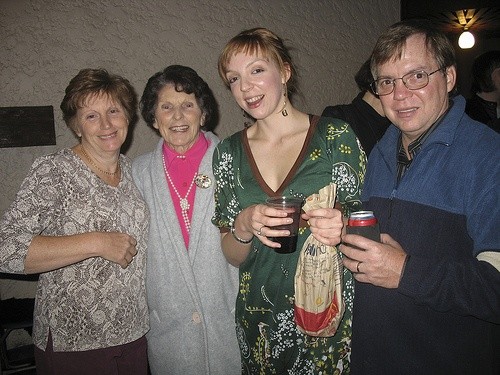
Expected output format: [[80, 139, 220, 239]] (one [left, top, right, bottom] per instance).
[[80, 145, 120, 175], [162, 154, 197, 234]]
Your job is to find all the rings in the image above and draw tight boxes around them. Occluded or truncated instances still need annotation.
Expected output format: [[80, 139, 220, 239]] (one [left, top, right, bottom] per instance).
[[257, 225, 265, 236], [356, 262, 362, 272]]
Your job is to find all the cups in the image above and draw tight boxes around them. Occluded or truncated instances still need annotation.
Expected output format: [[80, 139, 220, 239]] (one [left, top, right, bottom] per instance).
[[346, 222, 380, 251], [265, 196, 302, 254]]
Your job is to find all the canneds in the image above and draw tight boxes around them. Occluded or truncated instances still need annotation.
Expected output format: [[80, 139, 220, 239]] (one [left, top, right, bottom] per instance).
[[348, 210, 377, 227]]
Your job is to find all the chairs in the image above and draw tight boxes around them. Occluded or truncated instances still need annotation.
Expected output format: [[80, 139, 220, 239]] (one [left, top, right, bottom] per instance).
[[0, 298, 36, 375]]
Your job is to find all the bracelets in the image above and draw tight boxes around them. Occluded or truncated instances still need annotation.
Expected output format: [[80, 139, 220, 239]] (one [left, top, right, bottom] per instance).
[[230, 221, 254, 244]]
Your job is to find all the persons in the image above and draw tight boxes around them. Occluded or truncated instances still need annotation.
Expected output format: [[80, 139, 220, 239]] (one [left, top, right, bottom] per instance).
[[0, 67, 151, 375], [320, 59, 392, 160], [338, 21, 500, 375], [468, 51, 500, 135], [210, 28, 368, 375], [132, 65, 241, 375]]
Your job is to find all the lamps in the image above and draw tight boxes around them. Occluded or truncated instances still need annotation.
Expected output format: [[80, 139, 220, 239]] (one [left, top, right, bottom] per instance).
[[455, 30, 475, 49]]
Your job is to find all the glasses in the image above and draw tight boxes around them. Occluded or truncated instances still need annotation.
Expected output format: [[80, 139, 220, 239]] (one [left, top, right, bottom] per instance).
[[369, 66, 445, 96]]
